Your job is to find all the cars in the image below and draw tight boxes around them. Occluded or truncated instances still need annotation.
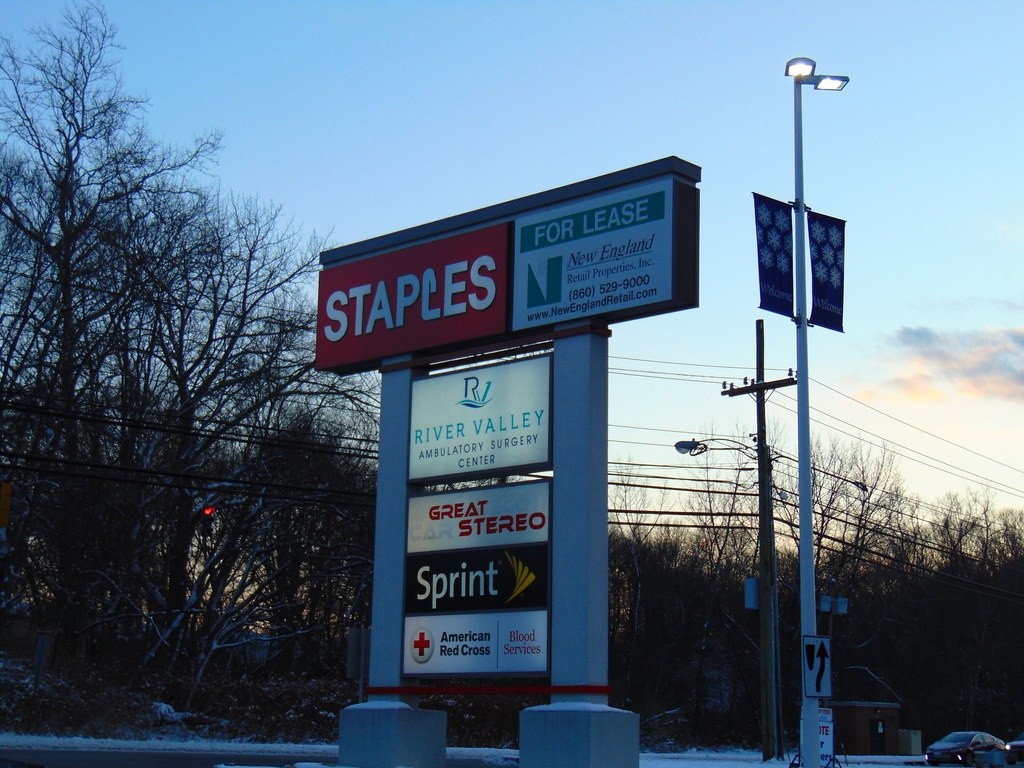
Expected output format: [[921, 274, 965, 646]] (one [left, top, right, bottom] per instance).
[[1005, 732, 1024, 765], [925, 731, 1005, 767]]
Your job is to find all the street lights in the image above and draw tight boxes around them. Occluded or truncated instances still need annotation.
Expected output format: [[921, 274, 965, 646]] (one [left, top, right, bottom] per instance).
[[785, 57, 851, 768], [674, 437, 868, 763]]
